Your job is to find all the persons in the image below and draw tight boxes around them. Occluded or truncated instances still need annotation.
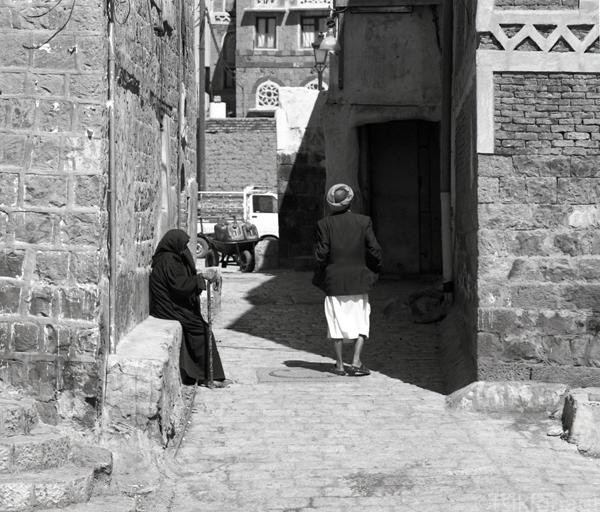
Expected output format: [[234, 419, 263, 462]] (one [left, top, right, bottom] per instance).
[[149, 228, 226, 387], [311, 183, 384, 375]]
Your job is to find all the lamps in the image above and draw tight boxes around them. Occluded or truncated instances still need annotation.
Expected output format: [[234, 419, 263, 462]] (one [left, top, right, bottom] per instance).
[[317, 4, 351, 57], [310, 30, 339, 92]]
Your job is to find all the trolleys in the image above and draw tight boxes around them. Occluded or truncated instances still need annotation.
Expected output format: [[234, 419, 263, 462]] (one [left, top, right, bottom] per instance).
[[200, 216, 261, 271]]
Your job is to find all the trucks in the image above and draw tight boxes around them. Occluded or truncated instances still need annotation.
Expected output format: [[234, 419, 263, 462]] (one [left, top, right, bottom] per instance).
[[197, 187, 279, 257]]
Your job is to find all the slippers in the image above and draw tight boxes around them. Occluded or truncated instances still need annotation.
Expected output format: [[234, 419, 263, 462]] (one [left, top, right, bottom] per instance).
[[330, 364, 370, 375]]
